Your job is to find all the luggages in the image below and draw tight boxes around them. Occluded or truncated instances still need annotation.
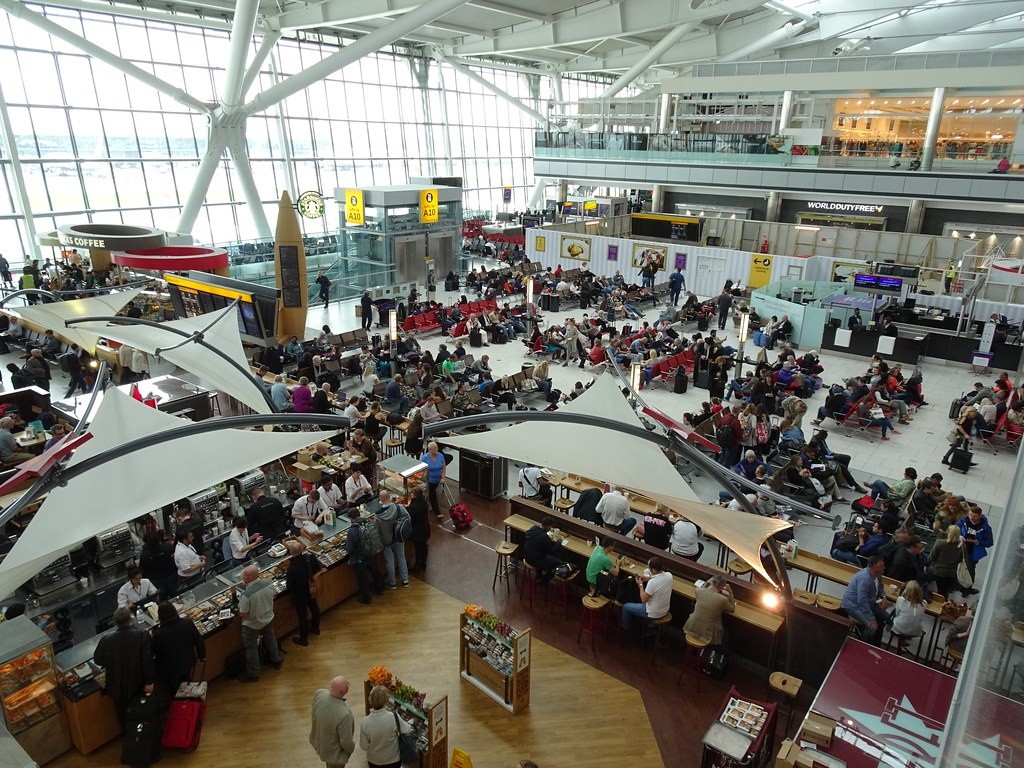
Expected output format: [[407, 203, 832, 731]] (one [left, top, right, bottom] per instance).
[[674, 358, 712, 394], [120, 657, 207, 768], [371, 332, 382, 348], [440, 482, 472, 529], [948, 437, 974, 474], [442, 315, 506, 347], [829, 510, 859, 558], [907, 366, 922, 394], [770, 416, 781, 448], [754, 323, 770, 348], [948, 392, 964, 419], [608, 319, 639, 339]]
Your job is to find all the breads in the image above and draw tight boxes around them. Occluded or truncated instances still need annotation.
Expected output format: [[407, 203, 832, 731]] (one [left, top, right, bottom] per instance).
[[260, 530, 348, 580], [0, 644, 52, 733], [396, 470, 424, 505], [187, 595, 229, 621]]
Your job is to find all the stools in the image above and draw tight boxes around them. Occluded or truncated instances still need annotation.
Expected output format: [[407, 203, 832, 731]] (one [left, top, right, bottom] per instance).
[[385, 439, 404, 459], [602, 523, 621, 534], [493, 498, 965, 742]]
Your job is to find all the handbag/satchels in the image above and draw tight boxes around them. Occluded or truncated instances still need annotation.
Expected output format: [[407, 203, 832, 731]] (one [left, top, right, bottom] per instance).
[[539, 486, 551, 498], [393, 712, 418, 764], [175, 678, 206, 701], [946, 434, 959, 444], [521, 379, 561, 403], [700, 648, 728, 681], [305, 552, 325, 598], [957, 546, 973, 592], [224, 645, 262, 680], [616, 578, 637, 603], [669, 273, 675, 288]]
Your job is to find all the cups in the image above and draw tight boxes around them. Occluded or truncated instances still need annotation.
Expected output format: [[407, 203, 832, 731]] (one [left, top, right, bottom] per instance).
[[890, 584, 897, 594], [586, 541, 592, 546], [204, 484, 238, 537], [80, 578, 89, 587]]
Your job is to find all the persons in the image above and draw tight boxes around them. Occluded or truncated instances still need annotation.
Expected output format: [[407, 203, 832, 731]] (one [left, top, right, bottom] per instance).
[[360, 686, 419, 768], [998, 157, 1011, 173], [309, 676, 355, 768], [0, 198, 1024, 734]]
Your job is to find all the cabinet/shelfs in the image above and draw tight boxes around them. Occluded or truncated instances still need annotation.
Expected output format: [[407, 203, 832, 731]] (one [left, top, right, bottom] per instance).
[[363, 678, 448, 768], [459, 612, 532, 716]]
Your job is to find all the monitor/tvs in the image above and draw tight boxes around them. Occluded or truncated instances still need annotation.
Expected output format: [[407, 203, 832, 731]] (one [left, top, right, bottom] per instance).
[[853, 324, 867, 331], [95, 538, 270, 635], [831, 318, 841, 328]]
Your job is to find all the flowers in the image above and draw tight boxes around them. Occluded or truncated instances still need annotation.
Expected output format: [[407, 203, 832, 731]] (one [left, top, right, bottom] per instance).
[[367, 664, 427, 708], [463, 603, 514, 640]]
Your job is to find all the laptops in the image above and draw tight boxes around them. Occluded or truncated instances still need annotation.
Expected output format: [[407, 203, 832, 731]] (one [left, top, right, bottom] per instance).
[[332, 391, 346, 405]]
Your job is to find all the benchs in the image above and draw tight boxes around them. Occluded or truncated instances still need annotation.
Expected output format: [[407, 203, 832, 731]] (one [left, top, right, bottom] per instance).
[[546, 473, 566, 513]]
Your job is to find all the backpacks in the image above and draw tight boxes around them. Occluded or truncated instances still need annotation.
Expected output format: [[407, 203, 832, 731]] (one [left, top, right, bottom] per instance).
[[717, 414, 769, 446], [593, 569, 619, 598], [353, 504, 410, 556], [259, 636, 281, 664]]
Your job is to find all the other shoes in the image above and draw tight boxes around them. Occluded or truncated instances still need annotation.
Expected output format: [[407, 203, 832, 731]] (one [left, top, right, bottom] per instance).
[[832, 482, 871, 505], [239, 663, 281, 682], [292, 626, 320, 646], [880, 416, 913, 441], [385, 581, 409, 590]]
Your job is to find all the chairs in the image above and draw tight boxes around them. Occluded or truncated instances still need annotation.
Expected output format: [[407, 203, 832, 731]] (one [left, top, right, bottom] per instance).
[[460, 237, 525, 259], [221, 234, 345, 264], [0, 320, 84, 471], [247, 263, 1024, 573], [509, 208, 557, 222]]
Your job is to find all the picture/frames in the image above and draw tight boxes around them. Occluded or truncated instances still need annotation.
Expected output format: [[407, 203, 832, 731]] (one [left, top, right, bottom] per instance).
[[536, 236, 877, 283]]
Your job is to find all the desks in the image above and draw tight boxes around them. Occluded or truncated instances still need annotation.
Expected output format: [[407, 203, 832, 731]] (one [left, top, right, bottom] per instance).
[[775, 633, 1024, 768], [559, 535, 633, 632], [699, 589, 785, 669], [620, 557, 698, 642], [559, 472, 725, 571], [769, 537, 818, 594], [998, 621, 1024, 690], [931, 606, 1014, 690], [502, 514, 570, 592], [810, 556, 946, 666], [11, 366, 467, 453]]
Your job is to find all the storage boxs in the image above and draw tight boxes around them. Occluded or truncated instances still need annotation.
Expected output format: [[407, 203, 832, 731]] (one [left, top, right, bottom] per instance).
[[293, 452, 326, 482], [774, 711, 836, 768]]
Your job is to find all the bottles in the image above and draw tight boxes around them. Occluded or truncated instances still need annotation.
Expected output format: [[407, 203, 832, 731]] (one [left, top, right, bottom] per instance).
[[135, 606, 145, 624]]
